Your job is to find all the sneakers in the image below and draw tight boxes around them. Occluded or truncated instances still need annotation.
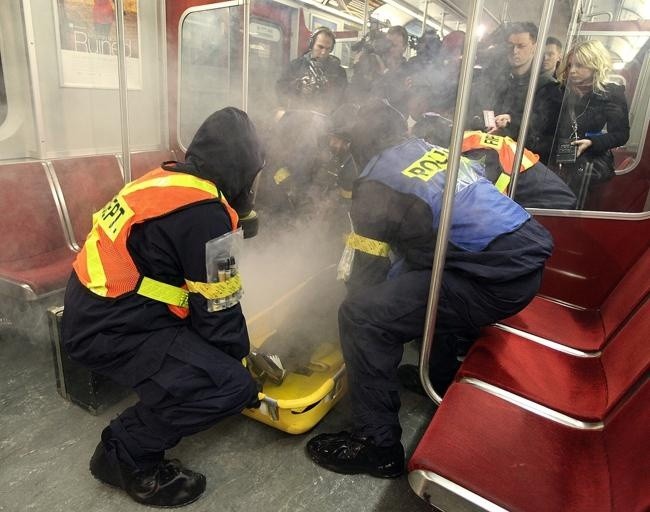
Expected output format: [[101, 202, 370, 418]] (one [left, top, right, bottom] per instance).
[[87, 428, 206, 507], [397, 364, 448, 399], [306, 428, 405, 479]]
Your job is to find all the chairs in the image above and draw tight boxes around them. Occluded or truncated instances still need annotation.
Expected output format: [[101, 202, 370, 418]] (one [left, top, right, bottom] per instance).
[[0, 143, 184, 297], [406, 153, 650, 511]]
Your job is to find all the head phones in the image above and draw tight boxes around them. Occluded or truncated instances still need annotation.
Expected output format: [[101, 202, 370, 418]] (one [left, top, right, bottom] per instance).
[[308, 28, 336, 52]]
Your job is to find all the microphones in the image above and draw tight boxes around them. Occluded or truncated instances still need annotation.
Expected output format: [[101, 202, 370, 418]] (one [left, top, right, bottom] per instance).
[[470, 110, 509, 132]]
